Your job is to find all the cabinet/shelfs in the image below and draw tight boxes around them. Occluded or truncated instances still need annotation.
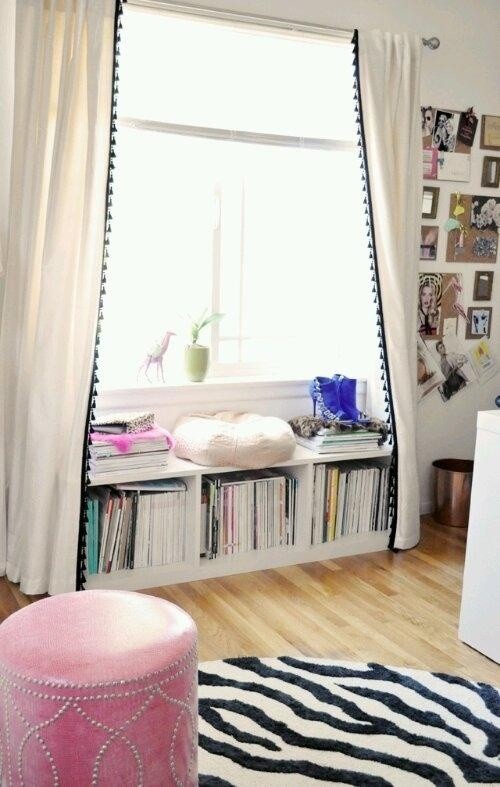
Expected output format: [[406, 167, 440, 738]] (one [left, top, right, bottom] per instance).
[[84, 445, 393, 589]]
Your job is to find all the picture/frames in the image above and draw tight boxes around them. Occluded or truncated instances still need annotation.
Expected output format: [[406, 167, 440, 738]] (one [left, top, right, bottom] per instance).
[[422, 186, 440, 219], [481, 156, 500, 188], [480, 115, 500, 150], [473, 271, 493, 300]]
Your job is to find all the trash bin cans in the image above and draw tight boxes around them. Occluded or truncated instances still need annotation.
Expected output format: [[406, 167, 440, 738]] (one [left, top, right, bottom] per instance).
[[431, 458, 473, 527]]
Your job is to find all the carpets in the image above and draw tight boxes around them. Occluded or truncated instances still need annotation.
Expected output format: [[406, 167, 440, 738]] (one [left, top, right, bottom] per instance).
[[198, 656, 500, 787]]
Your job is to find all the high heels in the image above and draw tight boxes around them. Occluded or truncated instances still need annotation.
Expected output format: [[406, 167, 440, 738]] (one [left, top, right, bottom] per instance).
[[308, 374, 367, 425]]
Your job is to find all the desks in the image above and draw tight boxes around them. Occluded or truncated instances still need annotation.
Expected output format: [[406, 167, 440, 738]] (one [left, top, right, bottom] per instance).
[[458, 409, 500, 666]]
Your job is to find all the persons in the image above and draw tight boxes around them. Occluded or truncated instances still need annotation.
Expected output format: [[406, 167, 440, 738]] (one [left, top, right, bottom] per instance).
[[434, 340, 470, 399], [418, 281, 441, 334], [421, 105, 437, 146], [442, 117, 460, 152], [433, 115, 448, 149]]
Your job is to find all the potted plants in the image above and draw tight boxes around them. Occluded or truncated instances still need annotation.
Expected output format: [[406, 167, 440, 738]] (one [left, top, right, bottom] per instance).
[[183, 312, 223, 382]]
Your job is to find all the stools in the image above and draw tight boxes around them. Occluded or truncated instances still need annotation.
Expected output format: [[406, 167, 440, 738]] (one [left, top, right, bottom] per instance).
[[0, 589, 199, 787]]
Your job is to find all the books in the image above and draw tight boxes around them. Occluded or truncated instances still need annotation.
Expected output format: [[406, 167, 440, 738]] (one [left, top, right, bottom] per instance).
[[200, 469, 299, 559], [312, 463, 389, 544], [86, 479, 186, 573], [87, 410, 172, 479], [292, 418, 381, 456]]
